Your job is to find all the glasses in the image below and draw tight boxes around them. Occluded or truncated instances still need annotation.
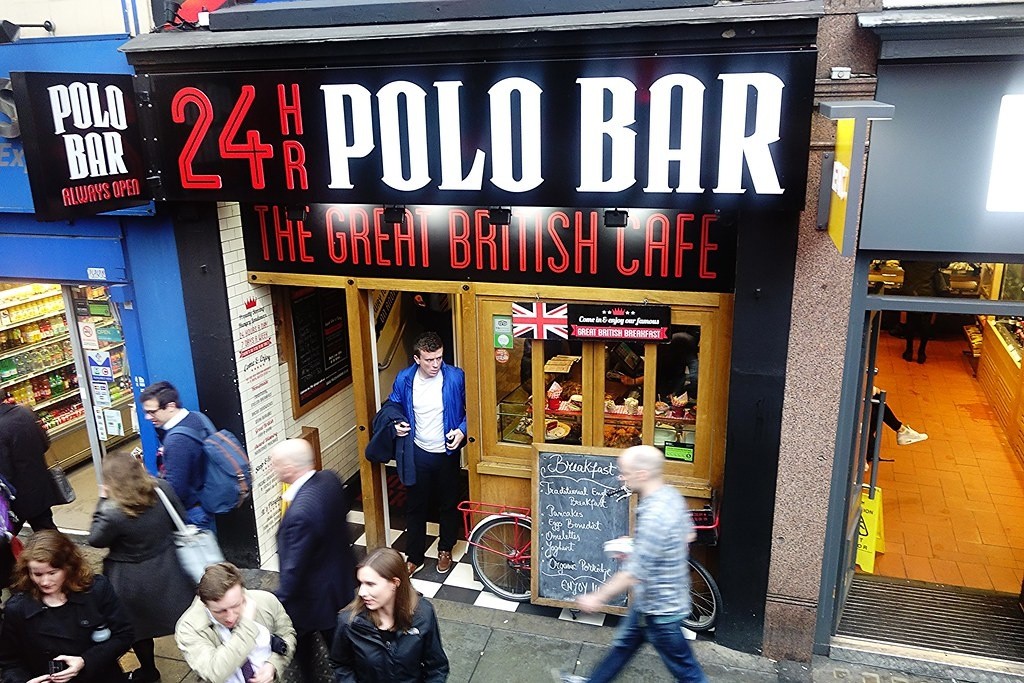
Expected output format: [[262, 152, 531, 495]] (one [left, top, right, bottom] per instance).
[[141, 408, 162, 414]]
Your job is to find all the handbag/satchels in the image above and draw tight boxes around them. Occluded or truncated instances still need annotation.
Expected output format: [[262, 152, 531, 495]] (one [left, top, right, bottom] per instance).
[[49, 467, 77, 506], [155, 487, 225, 582]]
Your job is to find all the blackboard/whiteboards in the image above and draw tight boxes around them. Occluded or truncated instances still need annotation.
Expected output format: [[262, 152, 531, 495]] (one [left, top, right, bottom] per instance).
[[531, 442, 637, 618]]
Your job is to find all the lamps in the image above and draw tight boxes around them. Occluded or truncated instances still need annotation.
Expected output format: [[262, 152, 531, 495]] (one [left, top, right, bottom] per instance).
[[382, 204, 407, 226], [285, 204, 308, 222], [603, 208, 629, 228], [0, 19, 55, 44], [489, 206, 513, 225]]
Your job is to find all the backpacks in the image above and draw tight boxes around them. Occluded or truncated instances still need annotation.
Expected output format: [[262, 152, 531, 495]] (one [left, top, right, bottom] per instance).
[[167, 411, 253, 513]]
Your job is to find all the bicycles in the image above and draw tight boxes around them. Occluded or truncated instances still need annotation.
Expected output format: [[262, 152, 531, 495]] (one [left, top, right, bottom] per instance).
[[457, 501, 722, 633]]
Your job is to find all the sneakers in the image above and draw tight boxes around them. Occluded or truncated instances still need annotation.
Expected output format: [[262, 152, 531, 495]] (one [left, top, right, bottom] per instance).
[[406, 561, 425, 579], [436, 551, 452, 573]]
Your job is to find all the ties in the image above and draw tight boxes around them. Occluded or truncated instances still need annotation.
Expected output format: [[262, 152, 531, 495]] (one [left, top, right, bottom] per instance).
[[229, 627, 254, 683]]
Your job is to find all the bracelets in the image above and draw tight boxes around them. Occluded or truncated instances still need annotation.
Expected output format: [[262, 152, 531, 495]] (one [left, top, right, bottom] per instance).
[[633, 377, 636, 386]]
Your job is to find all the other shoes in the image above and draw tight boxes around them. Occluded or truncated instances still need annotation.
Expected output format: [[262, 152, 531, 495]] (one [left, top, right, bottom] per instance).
[[917, 352, 926, 364], [551, 667, 592, 683], [128, 667, 162, 683], [902, 349, 913, 362], [896, 424, 928, 445]]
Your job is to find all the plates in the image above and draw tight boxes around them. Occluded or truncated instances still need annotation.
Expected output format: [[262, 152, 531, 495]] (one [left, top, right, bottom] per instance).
[[526, 421, 570, 440]]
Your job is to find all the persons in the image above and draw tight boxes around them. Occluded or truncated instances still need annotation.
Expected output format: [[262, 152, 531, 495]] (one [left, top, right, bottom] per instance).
[[329, 547, 450, 683], [864, 385, 928, 459], [550, 443, 708, 683], [899, 258, 952, 363], [139, 381, 216, 533], [386, 332, 468, 577], [620, 374, 645, 387], [267, 437, 356, 683], [173, 561, 298, 683], [0, 390, 62, 531], [87, 452, 197, 683], [0, 530, 149, 683]]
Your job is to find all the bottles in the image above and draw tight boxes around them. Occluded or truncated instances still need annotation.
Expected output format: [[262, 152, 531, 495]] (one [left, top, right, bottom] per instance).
[[8, 292, 65, 323], [70, 284, 104, 299], [105, 376, 133, 400], [0, 312, 73, 384], [109, 348, 124, 375], [0, 364, 79, 407]]
